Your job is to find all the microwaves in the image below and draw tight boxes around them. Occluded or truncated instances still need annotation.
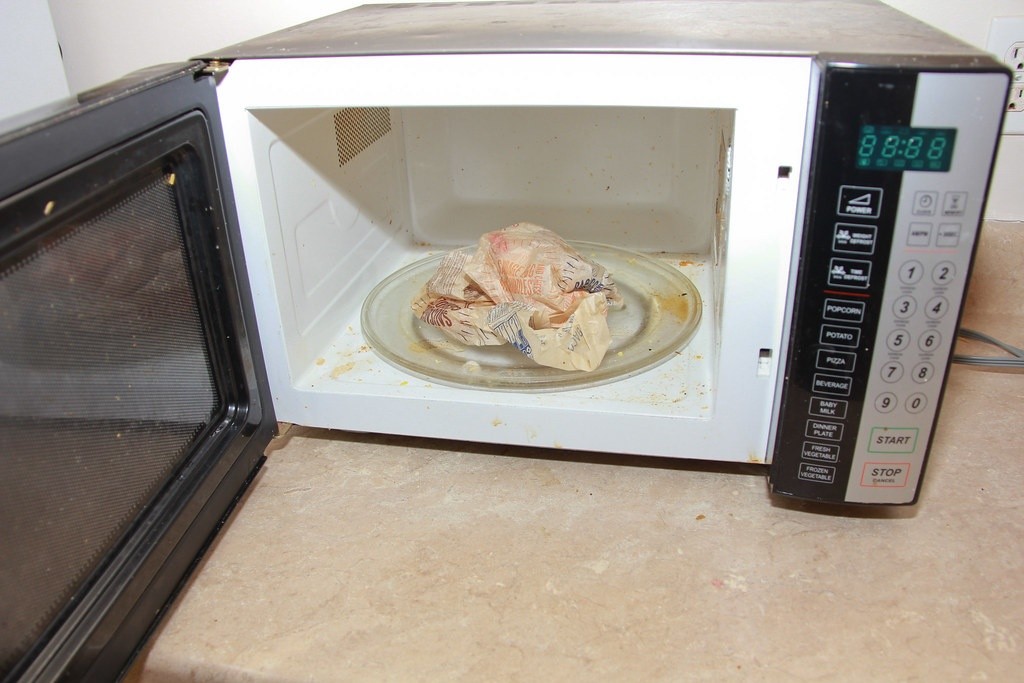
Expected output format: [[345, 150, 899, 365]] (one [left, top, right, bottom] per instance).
[[0, 0, 1014, 683]]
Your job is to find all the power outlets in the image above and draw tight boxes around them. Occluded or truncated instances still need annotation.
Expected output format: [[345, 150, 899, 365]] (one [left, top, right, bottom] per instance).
[[986, 17, 1024, 136]]
